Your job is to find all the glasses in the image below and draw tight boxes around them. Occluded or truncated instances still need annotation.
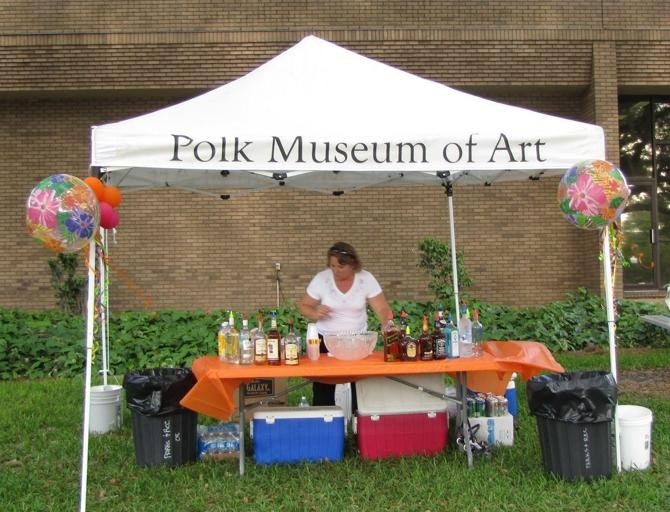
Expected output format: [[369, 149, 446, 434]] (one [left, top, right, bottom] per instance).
[[329, 247, 354, 256]]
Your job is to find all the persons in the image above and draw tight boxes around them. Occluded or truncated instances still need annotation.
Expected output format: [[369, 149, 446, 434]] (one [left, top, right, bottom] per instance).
[[301, 242, 394, 405]]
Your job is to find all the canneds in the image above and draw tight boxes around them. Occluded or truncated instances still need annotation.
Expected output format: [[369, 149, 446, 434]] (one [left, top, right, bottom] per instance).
[[467, 396, 508, 417]]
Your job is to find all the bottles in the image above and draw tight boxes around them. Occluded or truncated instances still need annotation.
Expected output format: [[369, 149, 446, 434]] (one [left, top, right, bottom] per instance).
[[197, 431, 240, 462], [299, 396, 311, 408], [217, 309, 320, 367], [382, 300, 484, 363]]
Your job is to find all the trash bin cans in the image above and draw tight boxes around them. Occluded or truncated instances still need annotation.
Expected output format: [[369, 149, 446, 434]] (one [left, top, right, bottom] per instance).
[[527, 371, 617, 482], [125, 367, 196, 467]]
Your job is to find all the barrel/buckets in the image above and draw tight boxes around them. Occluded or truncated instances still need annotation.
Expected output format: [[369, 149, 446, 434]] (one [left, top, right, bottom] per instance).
[[86, 384, 122, 435], [616, 403, 654, 472]]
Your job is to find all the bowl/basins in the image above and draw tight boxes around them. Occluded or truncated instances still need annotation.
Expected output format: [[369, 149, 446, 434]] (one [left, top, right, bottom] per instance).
[[323, 330, 379, 362]]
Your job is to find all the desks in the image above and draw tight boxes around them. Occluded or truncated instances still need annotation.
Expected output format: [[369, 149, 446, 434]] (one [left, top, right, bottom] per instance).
[[178, 340, 565, 478]]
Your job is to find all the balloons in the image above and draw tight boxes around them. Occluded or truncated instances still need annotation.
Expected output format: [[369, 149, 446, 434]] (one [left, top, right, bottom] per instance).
[[25, 174, 100, 253], [84, 176, 121, 229], [557, 158, 628, 230]]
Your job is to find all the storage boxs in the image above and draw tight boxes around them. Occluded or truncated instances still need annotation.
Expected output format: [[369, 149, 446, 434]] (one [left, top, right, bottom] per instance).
[[463, 414, 516, 449], [246, 405, 346, 466], [351, 371, 450, 460]]
